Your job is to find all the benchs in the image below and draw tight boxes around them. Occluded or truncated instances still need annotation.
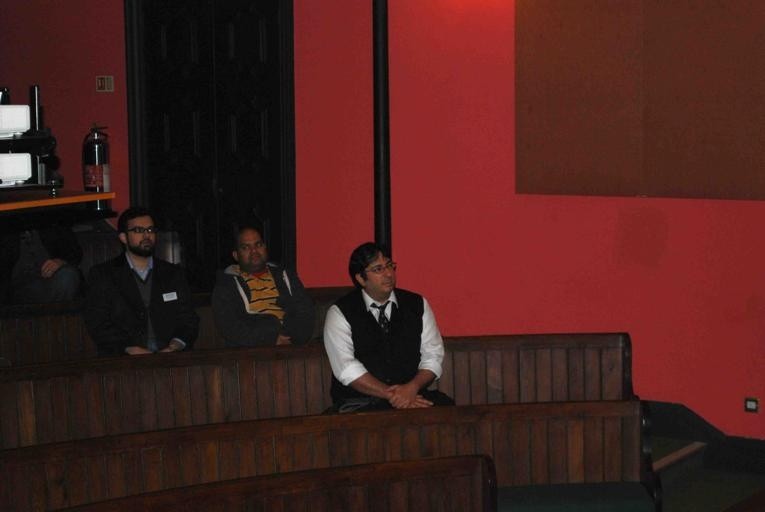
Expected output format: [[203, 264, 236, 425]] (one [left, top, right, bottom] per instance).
[[0, 301, 664, 512]]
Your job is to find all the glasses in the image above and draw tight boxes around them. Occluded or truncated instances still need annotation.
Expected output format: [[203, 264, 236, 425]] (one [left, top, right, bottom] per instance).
[[362, 260, 398, 275], [125, 225, 157, 234]]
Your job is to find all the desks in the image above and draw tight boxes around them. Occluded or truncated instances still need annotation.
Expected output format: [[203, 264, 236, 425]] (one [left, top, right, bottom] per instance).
[[0, 183, 116, 228]]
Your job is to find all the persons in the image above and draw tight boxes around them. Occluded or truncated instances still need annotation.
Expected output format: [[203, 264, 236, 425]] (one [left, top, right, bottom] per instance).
[[81, 205, 201, 358], [322, 243, 456, 415], [210, 226, 317, 347]]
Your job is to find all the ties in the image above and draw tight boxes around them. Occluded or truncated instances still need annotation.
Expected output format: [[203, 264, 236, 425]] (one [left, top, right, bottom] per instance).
[[371, 300, 391, 332]]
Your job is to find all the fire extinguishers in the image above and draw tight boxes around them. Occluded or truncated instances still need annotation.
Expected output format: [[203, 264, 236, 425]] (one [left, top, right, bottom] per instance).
[[82, 126, 118, 219]]
